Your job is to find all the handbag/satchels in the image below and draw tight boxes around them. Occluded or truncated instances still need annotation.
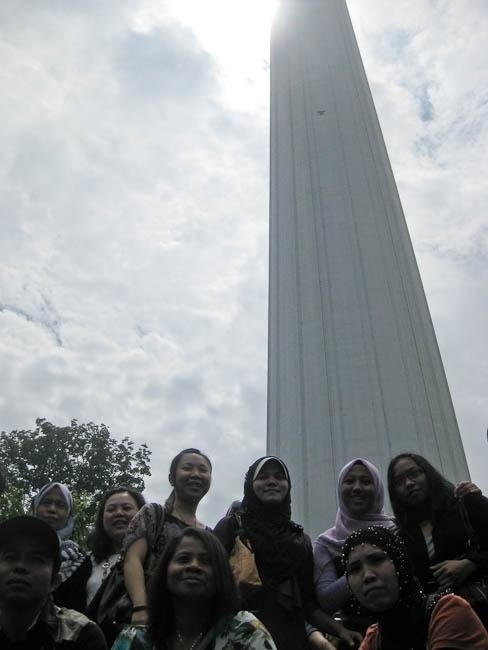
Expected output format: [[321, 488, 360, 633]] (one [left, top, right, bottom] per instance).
[[83, 561, 152, 641], [227, 536, 262, 601]]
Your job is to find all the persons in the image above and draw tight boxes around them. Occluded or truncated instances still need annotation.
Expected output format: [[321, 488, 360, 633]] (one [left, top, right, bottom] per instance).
[[26, 482, 92, 615], [341, 525, 488, 650], [0, 514, 108, 650], [212, 456, 315, 649], [118, 446, 214, 630], [314, 456, 394, 612], [82, 488, 143, 611], [389, 452, 488, 631], [110, 528, 279, 650]]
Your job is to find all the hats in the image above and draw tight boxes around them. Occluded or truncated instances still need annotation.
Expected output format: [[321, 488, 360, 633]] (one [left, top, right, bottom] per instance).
[[0, 516, 61, 570]]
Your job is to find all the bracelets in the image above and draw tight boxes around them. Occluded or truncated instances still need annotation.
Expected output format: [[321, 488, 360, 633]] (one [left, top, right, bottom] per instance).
[[130, 605, 149, 612]]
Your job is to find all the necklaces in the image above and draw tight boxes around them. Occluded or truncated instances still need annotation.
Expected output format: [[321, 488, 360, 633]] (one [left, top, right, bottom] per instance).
[[175, 506, 197, 528], [174, 628, 204, 649]]
